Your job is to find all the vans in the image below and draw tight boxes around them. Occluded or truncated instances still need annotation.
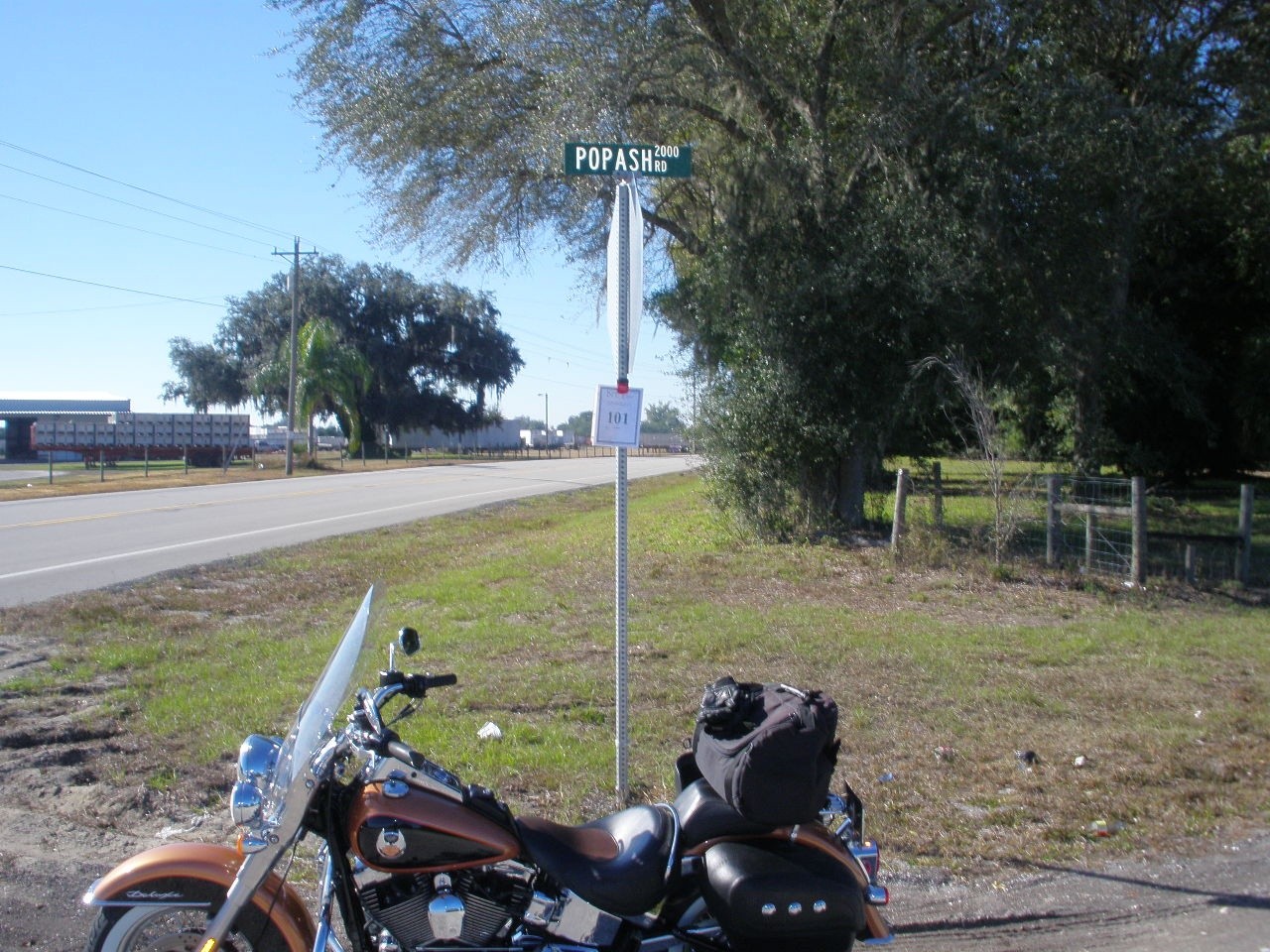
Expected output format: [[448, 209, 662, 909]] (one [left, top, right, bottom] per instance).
[[254, 439, 269, 452]]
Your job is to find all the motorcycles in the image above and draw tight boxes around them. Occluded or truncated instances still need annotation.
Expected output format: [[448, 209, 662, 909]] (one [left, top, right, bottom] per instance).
[[82, 580, 893, 952]]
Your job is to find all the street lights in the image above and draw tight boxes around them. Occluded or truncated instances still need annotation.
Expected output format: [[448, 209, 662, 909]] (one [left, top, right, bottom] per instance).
[[537, 393, 548, 454]]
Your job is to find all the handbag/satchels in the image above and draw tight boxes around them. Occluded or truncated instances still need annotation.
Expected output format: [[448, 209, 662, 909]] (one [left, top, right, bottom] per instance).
[[691, 673, 839, 824]]
